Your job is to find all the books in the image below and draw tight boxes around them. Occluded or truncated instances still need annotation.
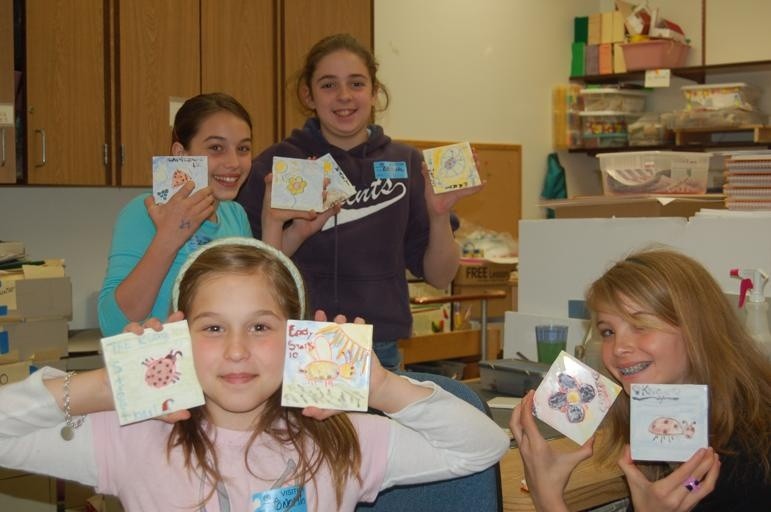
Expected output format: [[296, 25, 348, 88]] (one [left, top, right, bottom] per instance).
[[570, 11, 633, 75]]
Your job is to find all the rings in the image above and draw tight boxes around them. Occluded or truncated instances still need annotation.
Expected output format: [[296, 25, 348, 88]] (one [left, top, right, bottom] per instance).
[[684, 476, 701, 492]]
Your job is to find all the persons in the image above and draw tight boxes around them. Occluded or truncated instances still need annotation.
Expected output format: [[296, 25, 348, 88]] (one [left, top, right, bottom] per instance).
[[233, 30, 490, 373], [511, 242, 770, 510], [98, 91, 321, 337], [1, 236, 512, 511]]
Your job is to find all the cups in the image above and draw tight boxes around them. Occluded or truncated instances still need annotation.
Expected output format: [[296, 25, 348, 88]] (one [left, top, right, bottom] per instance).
[[536, 324, 570, 363]]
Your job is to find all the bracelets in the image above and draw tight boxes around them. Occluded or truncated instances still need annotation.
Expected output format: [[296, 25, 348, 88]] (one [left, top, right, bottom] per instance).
[[60, 368, 88, 441]]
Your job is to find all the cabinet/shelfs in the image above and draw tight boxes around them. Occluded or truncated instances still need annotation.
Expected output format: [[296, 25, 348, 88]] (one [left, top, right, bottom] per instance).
[[634, 0, 771, 67], [3, 1, 377, 189]]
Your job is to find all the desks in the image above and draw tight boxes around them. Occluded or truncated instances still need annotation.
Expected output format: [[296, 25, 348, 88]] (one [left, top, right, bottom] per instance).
[[390, 326, 500, 382], [491, 423, 660, 511]]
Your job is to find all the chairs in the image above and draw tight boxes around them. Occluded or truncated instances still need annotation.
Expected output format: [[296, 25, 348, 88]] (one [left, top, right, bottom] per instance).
[[359, 365, 508, 511]]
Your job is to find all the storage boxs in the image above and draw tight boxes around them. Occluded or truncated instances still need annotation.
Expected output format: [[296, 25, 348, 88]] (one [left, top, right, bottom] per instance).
[[399, 217, 519, 336], [594, 150, 716, 196], [551, 198, 726, 219], [481, 358, 554, 397], [576, 79, 763, 149], [622, 42, 692, 71]]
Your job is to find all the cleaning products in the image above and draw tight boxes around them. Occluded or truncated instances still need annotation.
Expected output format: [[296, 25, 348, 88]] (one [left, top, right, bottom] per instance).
[[730, 266, 771, 353]]
